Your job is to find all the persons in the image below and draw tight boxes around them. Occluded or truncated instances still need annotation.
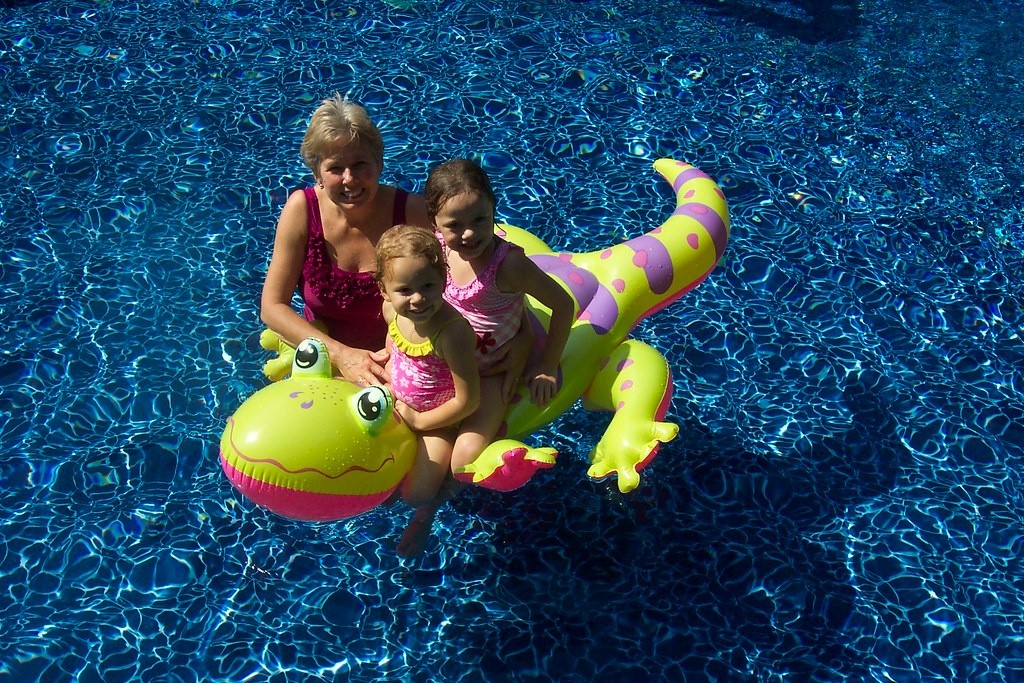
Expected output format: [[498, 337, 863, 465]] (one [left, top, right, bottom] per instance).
[[376, 157, 576, 507], [375, 224, 481, 564], [258, 92, 535, 407]]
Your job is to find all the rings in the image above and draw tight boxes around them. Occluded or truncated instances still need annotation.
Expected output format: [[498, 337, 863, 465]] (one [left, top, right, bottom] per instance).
[[515, 377, 520, 381]]
